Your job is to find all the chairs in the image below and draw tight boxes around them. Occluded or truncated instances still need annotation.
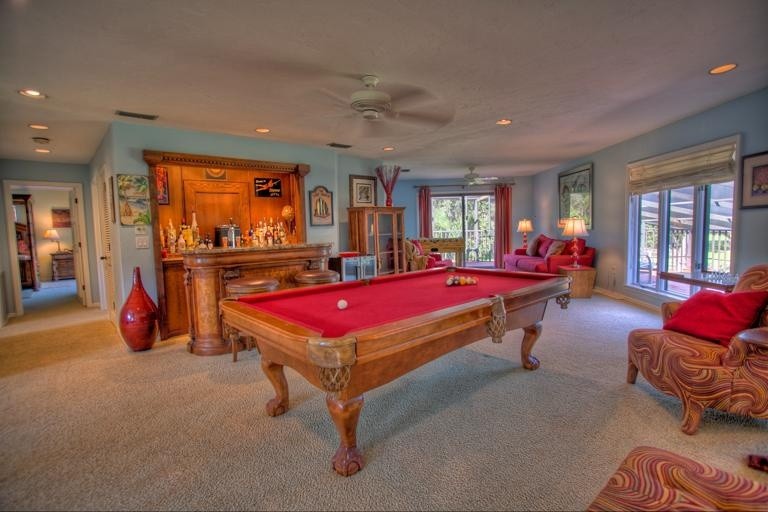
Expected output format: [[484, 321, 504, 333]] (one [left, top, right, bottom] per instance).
[[406, 237, 452, 273], [626, 263, 767, 437]]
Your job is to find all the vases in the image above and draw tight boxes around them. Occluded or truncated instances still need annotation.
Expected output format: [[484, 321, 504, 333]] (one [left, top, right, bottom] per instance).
[[386, 197, 393, 207], [120, 267, 157, 353]]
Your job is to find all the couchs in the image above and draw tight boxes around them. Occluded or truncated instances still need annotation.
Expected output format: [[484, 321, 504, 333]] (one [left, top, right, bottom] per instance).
[[501, 234, 596, 272]]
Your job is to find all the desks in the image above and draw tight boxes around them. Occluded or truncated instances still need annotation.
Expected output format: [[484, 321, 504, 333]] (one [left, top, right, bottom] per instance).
[[216, 263, 573, 477], [413, 238, 466, 267], [655, 269, 736, 294], [558, 265, 596, 298]]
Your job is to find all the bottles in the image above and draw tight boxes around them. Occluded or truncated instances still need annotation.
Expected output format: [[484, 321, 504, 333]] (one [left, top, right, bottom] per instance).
[[228, 218, 235, 247], [160, 211, 213, 252], [241, 217, 285, 246]]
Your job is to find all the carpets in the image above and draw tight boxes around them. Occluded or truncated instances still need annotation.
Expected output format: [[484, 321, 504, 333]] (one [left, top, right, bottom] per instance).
[[1, 344, 768, 511]]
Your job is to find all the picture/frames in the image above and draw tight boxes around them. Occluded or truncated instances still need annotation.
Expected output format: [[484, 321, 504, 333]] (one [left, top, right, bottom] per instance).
[[309, 186, 334, 228], [108, 175, 116, 225], [50, 208, 71, 230], [556, 161, 594, 230], [349, 174, 376, 207], [739, 149, 767, 210]]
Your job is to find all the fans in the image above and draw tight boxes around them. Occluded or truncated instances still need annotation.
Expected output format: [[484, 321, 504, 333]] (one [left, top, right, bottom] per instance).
[[445, 162, 500, 186], [303, 74, 455, 148]]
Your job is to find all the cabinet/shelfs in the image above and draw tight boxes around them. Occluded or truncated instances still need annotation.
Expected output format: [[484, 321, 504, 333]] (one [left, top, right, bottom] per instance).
[[50, 249, 75, 282], [140, 150, 313, 345], [10, 192, 38, 294], [346, 205, 406, 276], [329, 256, 378, 282]]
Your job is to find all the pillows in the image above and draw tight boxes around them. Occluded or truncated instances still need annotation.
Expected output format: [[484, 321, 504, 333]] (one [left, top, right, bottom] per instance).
[[525, 234, 586, 260], [662, 288, 767, 347]]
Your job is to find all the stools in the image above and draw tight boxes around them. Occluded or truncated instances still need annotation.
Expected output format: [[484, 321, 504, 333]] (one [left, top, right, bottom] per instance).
[[224, 276, 278, 362], [294, 269, 342, 287], [583, 446, 767, 512]]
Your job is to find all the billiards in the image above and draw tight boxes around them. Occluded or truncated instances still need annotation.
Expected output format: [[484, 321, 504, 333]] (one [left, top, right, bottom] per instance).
[[338, 300, 348, 309], [446, 275, 479, 286]]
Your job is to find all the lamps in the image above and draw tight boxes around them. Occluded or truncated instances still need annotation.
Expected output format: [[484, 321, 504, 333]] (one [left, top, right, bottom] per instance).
[[43, 229, 63, 254], [564, 216, 585, 269], [516, 219, 534, 248]]
